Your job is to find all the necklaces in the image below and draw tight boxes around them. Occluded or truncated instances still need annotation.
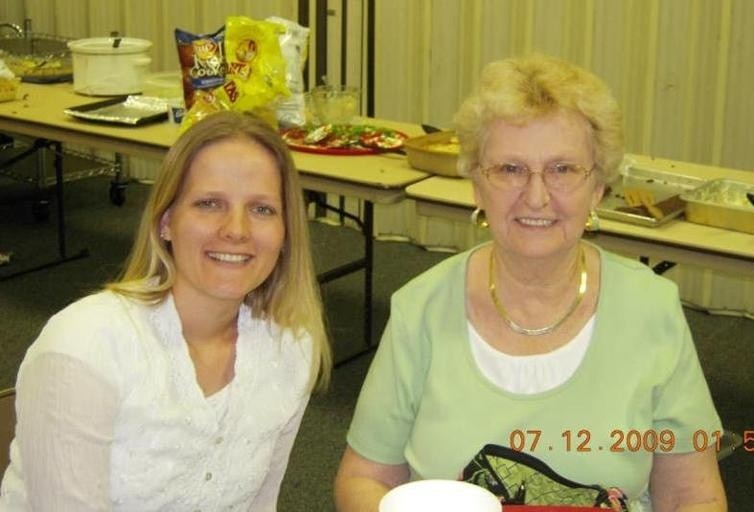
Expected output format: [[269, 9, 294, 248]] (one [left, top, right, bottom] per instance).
[[488, 247, 589, 334]]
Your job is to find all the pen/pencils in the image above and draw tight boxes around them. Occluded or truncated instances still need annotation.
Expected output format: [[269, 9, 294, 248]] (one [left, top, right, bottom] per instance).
[[517, 480, 526, 504]]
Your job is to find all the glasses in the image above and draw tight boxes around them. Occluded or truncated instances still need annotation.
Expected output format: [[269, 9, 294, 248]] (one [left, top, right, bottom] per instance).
[[477, 160, 597, 193]]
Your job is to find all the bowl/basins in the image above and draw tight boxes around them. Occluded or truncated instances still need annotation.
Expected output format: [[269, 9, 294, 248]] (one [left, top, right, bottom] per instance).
[[67, 36, 153, 98]]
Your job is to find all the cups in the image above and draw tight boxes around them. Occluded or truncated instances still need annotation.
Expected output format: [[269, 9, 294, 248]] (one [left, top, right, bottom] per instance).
[[377, 478, 505, 512], [302, 84, 362, 127]]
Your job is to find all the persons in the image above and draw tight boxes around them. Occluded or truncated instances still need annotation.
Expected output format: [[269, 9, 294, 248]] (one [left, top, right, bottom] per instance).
[[330, 57, 730, 512], [0, 110, 330, 511]]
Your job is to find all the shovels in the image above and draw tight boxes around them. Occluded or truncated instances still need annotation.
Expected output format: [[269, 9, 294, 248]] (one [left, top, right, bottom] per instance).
[[623, 185, 663, 220]]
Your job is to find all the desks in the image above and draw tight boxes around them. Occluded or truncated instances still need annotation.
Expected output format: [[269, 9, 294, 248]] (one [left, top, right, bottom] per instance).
[[404, 148, 754, 283], [0, 64, 450, 379]]
